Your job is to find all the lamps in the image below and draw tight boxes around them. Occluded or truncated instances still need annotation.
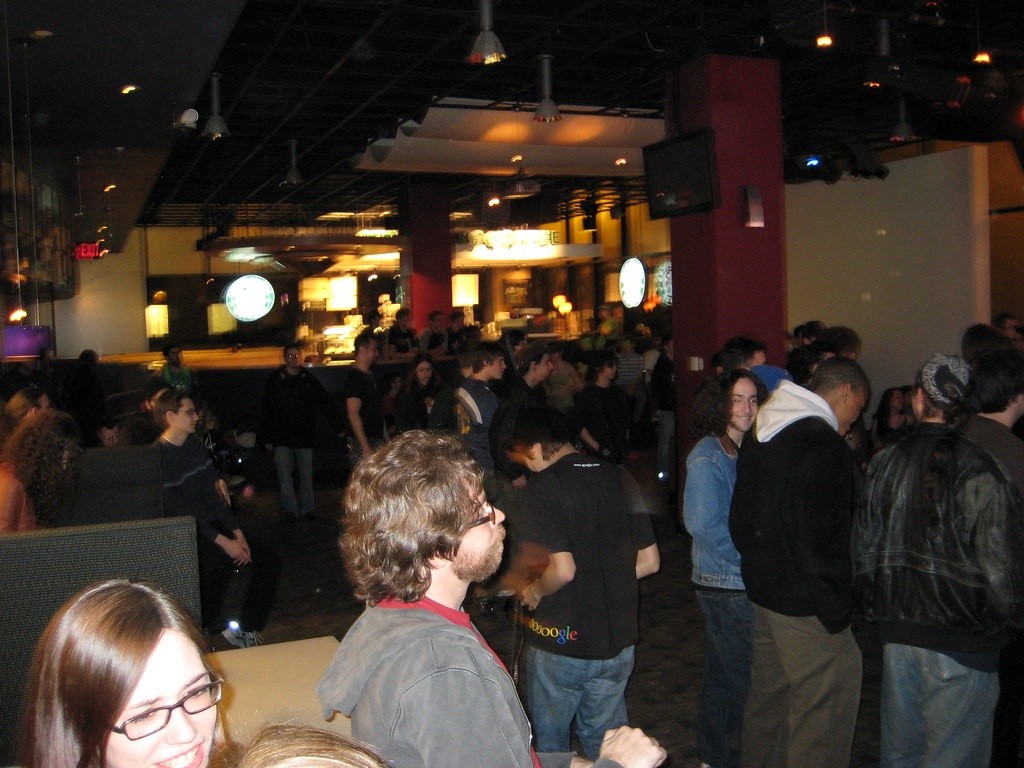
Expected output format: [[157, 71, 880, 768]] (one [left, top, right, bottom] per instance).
[[470, 0, 506, 66], [0, 0, 52, 359], [200, 73, 232, 141], [864, 19, 891, 90], [888, 93, 921, 143], [286, 139, 304, 185], [534, 54, 562, 122]]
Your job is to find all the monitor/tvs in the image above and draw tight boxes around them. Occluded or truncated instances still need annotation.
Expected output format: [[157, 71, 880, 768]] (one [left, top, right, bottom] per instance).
[[643, 126, 722, 220]]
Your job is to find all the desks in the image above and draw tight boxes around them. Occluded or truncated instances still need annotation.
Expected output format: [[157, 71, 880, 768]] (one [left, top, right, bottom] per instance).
[[202, 633, 352, 748]]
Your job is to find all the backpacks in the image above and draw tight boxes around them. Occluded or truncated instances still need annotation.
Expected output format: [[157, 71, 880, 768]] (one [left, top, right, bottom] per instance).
[[649, 351, 675, 404]]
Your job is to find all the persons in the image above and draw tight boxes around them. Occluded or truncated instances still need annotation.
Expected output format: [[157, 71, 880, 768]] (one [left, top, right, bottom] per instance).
[[0, 301, 1024, 768]]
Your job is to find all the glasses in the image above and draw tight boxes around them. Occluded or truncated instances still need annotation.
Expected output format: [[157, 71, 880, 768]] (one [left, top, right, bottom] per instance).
[[112, 662, 225, 741], [466, 501, 495, 530], [286, 354, 300, 358]]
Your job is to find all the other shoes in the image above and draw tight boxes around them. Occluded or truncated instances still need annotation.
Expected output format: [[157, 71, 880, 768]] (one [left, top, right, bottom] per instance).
[[280, 511, 296, 526], [305, 510, 324, 520], [221, 621, 263, 648]]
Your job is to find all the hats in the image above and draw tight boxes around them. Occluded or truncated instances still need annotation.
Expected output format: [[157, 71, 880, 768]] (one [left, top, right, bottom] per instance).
[[918, 351, 972, 413]]
